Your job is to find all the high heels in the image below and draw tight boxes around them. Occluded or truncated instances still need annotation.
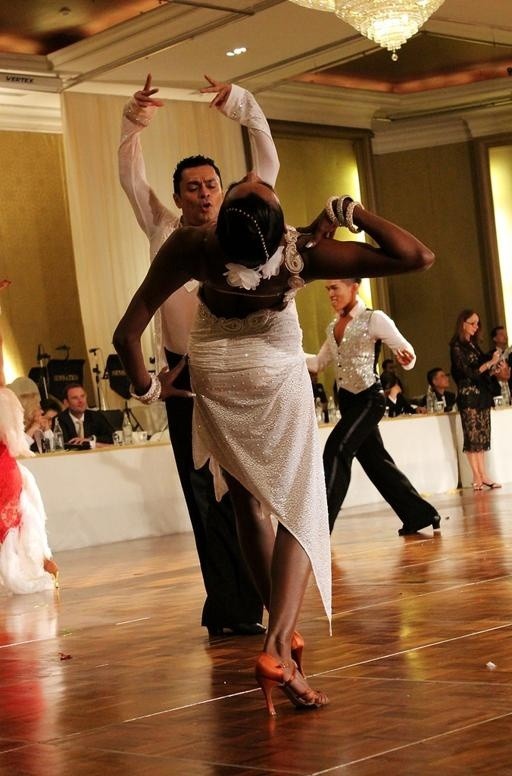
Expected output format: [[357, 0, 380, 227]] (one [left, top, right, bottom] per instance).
[[254, 652, 332, 716], [290, 630, 306, 680]]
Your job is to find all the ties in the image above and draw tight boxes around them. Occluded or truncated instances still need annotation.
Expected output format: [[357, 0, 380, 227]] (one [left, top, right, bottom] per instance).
[[76, 420, 84, 439]]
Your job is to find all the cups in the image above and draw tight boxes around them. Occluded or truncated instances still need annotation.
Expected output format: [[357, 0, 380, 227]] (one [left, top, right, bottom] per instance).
[[112, 432, 119, 447], [492, 396, 503, 408], [133, 432, 148, 444], [436, 401, 444, 414], [384, 406, 390, 419]]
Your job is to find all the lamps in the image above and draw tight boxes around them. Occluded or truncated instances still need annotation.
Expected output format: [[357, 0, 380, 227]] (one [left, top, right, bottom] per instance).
[[289, 0, 444, 61]]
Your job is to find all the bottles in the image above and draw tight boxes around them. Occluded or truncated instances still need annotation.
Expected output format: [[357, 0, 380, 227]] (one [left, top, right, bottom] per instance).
[[327, 396, 337, 423], [426, 384, 433, 416], [316, 396, 323, 427], [122, 412, 133, 444], [500, 377, 510, 407], [54, 417, 64, 451]]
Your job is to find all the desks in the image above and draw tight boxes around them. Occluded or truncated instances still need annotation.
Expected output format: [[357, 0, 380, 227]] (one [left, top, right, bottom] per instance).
[[445, 406, 511, 489], [13, 407, 461, 550]]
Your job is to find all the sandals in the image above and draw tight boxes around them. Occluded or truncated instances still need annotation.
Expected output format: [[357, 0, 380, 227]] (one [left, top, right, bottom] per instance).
[[482, 481, 502, 488], [471, 481, 483, 490]]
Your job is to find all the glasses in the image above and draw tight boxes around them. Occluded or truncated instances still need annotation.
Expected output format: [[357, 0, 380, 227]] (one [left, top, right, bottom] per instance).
[[465, 321, 476, 327]]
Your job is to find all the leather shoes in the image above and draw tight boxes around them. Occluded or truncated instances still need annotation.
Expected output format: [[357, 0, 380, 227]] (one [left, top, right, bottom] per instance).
[[206, 622, 267, 637], [398, 515, 441, 536]]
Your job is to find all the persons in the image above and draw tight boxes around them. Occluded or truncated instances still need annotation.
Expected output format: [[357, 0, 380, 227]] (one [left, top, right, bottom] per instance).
[[8, 377, 115, 454], [447, 307, 511, 494], [116, 71, 278, 636], [304, 278, 441, 539], [110, 167, 437, 715], [484, 325, 511, 391], [1, 385, 61, 594], [380, 359, 457, 418], [309, 371, 330, 423]]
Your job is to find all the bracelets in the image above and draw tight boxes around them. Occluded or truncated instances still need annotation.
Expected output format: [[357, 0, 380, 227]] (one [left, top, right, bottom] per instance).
[[346, 201, 365, 233], [337, 195, 354, 228], [486, 360, 493, 369], [324, 196, 343, 227], [129, 372, 162, 404]]
